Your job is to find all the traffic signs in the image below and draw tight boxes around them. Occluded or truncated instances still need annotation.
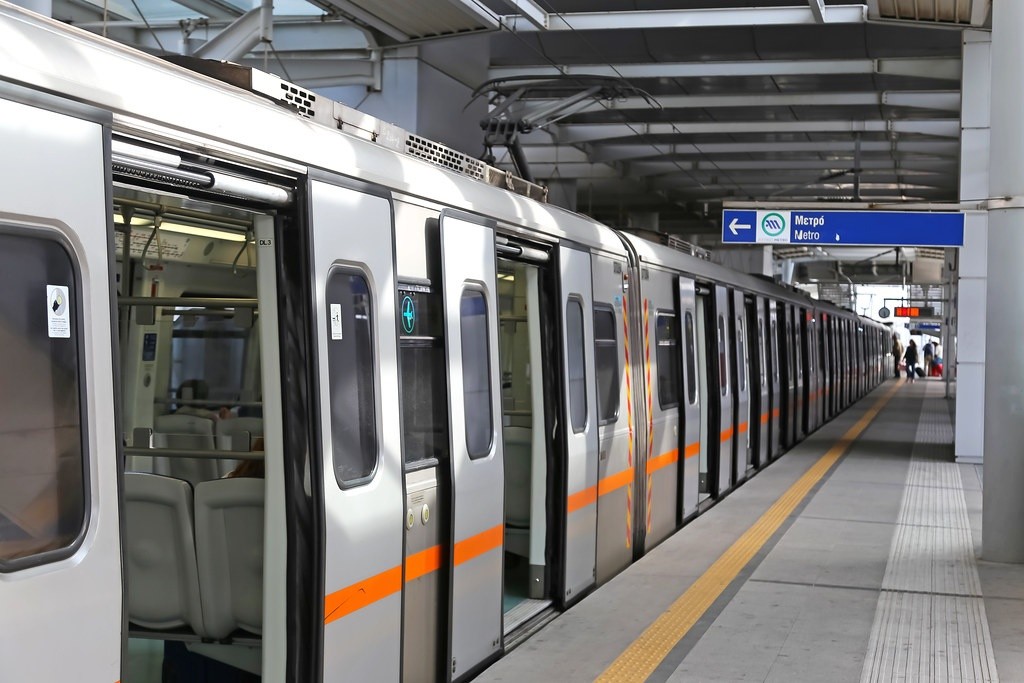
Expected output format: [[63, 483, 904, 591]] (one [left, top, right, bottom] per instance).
[[722, 208, 966, 248]]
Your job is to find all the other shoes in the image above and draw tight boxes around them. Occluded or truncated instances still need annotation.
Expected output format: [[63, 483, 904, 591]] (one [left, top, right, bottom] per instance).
[[906, 377, 910, 382], [911, 380, 914, 383]]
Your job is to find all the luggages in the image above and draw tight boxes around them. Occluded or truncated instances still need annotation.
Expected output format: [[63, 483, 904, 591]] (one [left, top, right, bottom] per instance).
[[915, 367, 926, 377]]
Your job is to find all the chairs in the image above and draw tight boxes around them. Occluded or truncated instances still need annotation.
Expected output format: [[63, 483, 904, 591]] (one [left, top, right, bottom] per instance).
[[504, 423, 533, 532], [124, 410, 268, 643]]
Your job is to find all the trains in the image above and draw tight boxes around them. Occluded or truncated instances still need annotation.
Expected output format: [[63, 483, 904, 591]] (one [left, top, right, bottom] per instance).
[[0, 1, 896, 683]]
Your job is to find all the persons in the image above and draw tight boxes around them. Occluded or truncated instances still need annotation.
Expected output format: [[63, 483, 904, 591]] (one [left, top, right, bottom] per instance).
[[219, 438, 265, 479], [922, 337, 941, 377], [891, 334, 903, 378], [901, 338, 918, 383], [174, 377, 240, 422]]
